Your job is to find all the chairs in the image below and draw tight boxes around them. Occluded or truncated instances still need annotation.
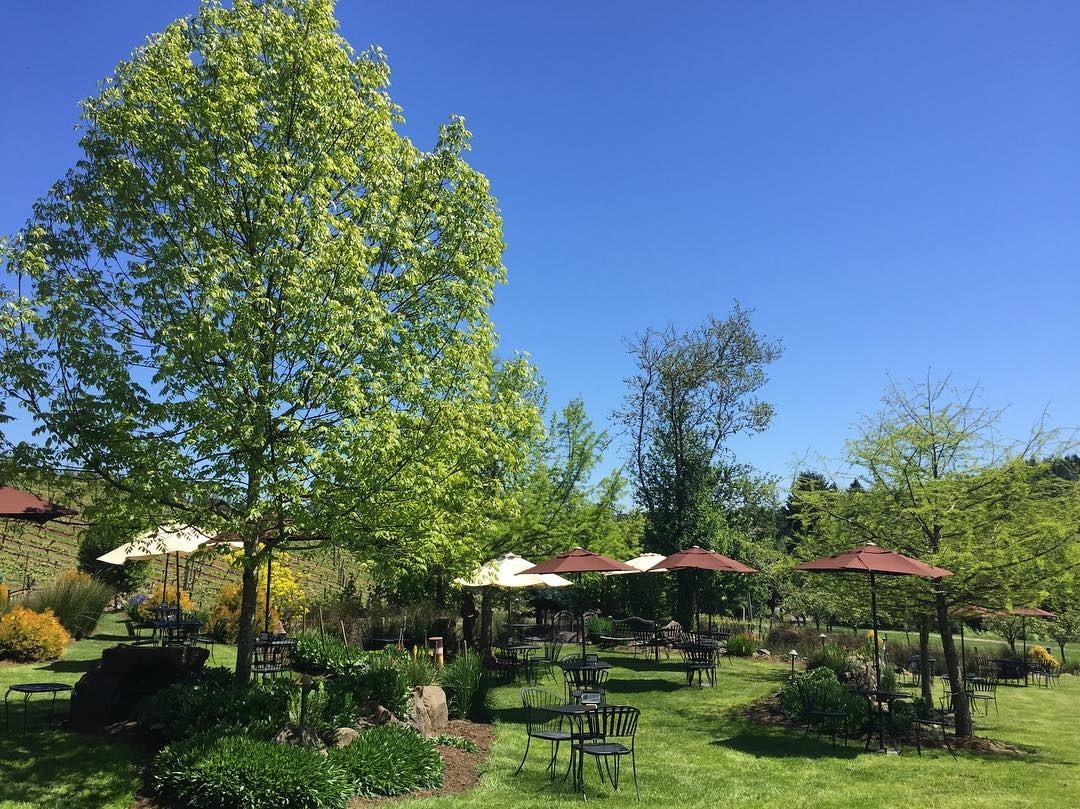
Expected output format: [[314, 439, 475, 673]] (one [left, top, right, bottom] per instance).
[[495, 617, 733, 801], [913, 652, 1062, 762], [796, 676, 847, 748], [116, 604, 232, 666]]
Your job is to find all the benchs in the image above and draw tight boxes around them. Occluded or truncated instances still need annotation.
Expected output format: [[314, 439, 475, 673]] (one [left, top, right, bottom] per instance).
[[253, 639, 300, 680], [598, 618, 656, 653]]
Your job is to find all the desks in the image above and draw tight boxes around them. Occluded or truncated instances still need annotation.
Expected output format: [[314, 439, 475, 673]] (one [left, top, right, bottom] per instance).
[[848, 689, 913, 758], [153, 621, 202, 642], [908, 658, 937, 687], [540, 705, 634, 784], [942, 676, 984, 714], [670, 645, 721, 684], [689, 631, 732, 653], [145, 607, 178, 621], [557, 660, 612, 702], [497, 643, 542, 683], [5, 682, 74, 737], [989, 657, 1027, 686]]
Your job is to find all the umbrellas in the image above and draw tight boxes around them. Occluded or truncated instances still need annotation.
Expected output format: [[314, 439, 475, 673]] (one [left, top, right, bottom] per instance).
[[0, 483, 78, 524], [793, 543, 954, 746], [512, 547, 643, 690], [596, 552, 684, 660], [644, 545, 757, 687], [96, 507, 335, 665], [449, 552, 573, 679], [948, 605, 1056, 691]]
